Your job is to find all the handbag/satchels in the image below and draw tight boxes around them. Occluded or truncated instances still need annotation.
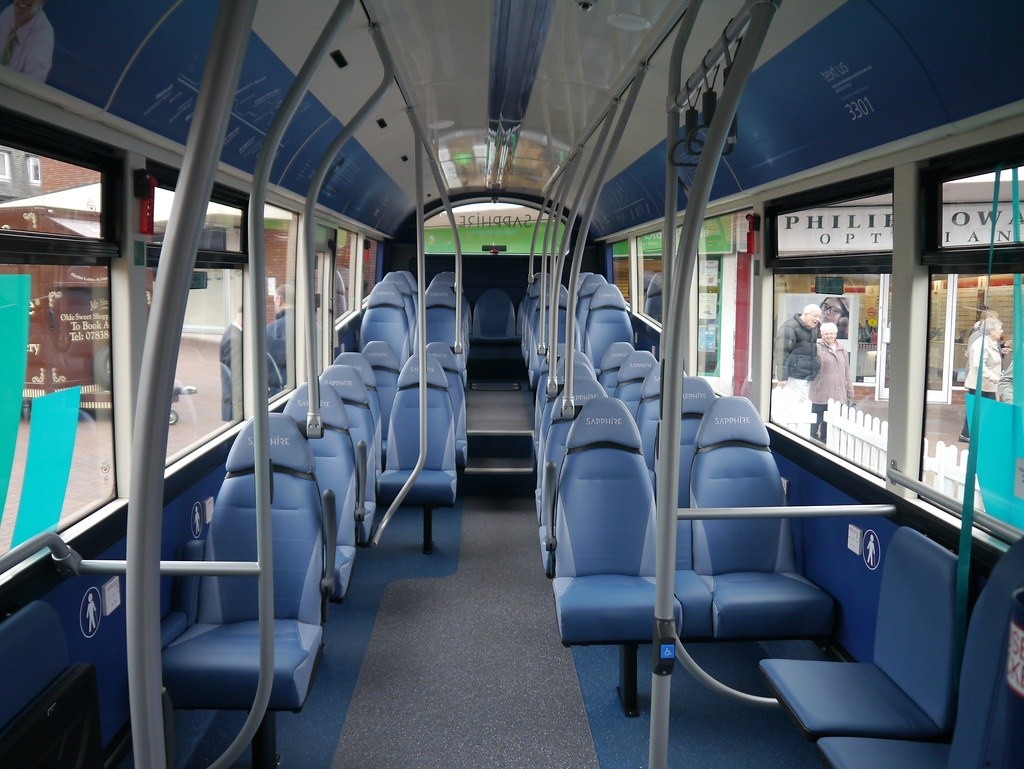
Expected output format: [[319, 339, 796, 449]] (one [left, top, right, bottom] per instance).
[[771, 386, 797, 423]]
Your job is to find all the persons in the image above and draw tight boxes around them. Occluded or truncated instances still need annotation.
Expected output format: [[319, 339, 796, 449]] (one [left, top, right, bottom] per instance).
[[219, 304, 242, 421], [266, 283, 294, 396], [808, 322, 855, 443], [816, 297, 849, 339], [771, 304, 823, 438], [957, 309, 1012, 442]]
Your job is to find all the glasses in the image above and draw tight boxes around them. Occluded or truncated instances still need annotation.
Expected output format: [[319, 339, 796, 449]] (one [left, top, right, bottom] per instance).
[[821, 303, 844, 315]]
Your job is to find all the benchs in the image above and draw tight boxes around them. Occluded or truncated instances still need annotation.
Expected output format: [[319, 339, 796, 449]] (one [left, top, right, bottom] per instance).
[[329, 266, 1024, 769]]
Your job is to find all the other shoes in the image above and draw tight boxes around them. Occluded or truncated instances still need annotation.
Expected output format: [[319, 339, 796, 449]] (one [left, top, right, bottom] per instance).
[[959, 435, 970, 443]]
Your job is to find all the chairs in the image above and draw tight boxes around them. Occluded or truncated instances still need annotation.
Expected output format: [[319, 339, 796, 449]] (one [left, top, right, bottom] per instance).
[[158, 364, 378, 768]]
